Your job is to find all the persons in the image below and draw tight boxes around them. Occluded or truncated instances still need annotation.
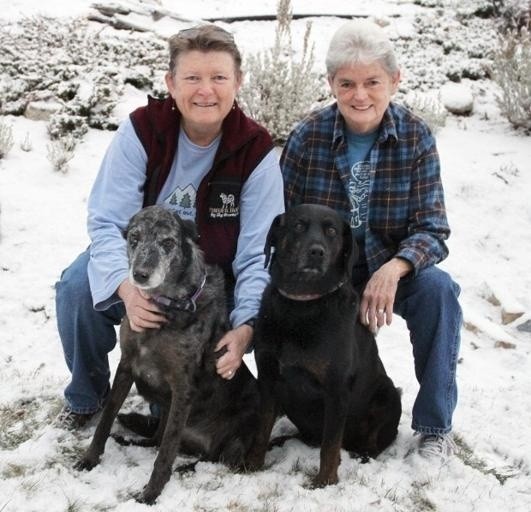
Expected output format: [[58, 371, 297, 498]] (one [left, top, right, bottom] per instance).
[[283, 20, 464, 473], [53, 26, 285, 440]]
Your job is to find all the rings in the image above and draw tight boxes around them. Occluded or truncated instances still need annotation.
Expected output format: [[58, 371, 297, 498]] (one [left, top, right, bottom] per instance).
[[227, 370, 234, 376], [377, 309, 385, 314]]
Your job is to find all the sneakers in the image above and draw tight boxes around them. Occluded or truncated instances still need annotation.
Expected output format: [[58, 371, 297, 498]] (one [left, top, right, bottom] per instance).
[[49, 392, 111, 435], [406, 425, 459, 486]]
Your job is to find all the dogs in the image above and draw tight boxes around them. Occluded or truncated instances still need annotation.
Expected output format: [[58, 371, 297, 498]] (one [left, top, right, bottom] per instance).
[[71, 204, 260, 506], [246, 204, 403, 491]]
